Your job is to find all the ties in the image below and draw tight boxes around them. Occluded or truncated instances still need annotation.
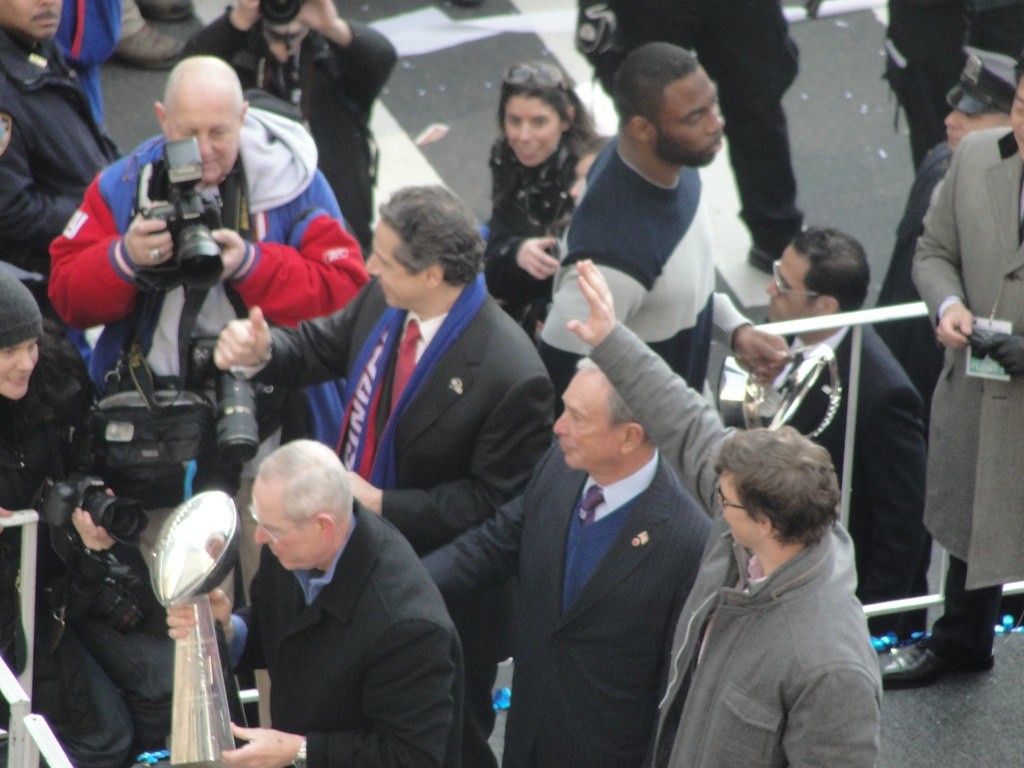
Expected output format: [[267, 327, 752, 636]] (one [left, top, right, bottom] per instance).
[[578, 485, 604, 527], [391, 320, 421, 418], [789, 352, 804, 374]]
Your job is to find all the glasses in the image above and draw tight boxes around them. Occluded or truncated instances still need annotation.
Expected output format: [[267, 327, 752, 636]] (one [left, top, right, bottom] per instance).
[[772, 259, 818, 297], [502, 64, 569, 91], [249, 505, 335, 544], [716, 488, 744, 509]]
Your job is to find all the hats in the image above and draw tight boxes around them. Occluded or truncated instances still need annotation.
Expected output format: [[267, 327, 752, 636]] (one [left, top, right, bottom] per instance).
[[946, 44, 1019, 114], [0, 269, 43, 347]]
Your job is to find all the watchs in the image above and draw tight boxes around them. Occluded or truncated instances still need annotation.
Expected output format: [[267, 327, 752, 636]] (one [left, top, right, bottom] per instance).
[[292, 737, 307, 768]]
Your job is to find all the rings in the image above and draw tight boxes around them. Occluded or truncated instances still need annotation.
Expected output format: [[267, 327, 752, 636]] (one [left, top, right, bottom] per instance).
[[150, 249, 160, 260]]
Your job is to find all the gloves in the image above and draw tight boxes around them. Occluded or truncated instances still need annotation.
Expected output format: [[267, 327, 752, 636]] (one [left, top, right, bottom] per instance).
[[967, 329, 1024, 375]]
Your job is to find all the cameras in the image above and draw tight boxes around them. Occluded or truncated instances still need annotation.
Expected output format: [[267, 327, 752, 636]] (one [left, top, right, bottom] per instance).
[[144, 138, 226, 286], [967, 329, 994, 360], [260, 0, 305, 24], [44, 566, 146, 636], [189, 335, 259, 463], [31, 469, 149, 546]]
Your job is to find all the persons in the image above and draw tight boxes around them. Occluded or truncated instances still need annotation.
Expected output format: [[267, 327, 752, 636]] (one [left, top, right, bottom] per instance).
[[0, 0, 1024, 768]]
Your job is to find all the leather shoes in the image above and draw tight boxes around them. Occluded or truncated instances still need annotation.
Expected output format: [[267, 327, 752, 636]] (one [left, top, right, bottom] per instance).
[[882, 640, 995, 690]]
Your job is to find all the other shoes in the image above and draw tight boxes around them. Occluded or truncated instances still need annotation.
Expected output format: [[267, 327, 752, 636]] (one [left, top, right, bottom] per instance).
[[112, 26, 187, 71], [748, 248, 778, 275]]
